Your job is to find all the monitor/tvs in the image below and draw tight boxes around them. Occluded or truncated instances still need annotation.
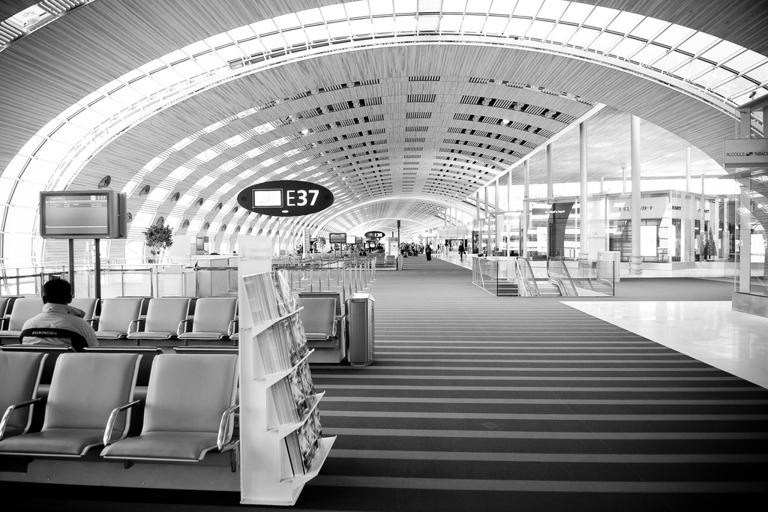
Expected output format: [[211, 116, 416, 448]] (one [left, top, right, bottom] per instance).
[[329, 233, 348, 244], [117, 192, 128, 239], [39, 189, 119, 239]]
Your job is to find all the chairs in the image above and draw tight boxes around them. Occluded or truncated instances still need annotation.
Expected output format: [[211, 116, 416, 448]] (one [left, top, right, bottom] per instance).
[[0, 352, 49, 440], [0, 352, 144, 470], [100, 352, 239, 472], [1, 292, 339, 342], [1, 342, 239, 386]]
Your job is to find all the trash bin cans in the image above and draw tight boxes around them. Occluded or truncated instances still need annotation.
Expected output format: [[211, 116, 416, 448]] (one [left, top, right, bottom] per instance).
[[397, 254, 403, 271], [349, 293, 375, 367]]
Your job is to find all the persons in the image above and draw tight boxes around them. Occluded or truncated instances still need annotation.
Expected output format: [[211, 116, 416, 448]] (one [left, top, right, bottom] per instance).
[[458, 244, 465, 260], [295, 248, 301, 254], [424, 243, 431, 264], [358, 249, 366, 256], [509, 250, 517, 256], [326, 246, 334, 253], [375, 243, 383, 253], [399, 240, 423, 259], [18, 278, 101, 348]]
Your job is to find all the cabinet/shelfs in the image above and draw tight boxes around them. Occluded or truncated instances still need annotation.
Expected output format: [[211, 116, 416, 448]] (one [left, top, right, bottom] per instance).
[[233, 270, 338, 507]]
